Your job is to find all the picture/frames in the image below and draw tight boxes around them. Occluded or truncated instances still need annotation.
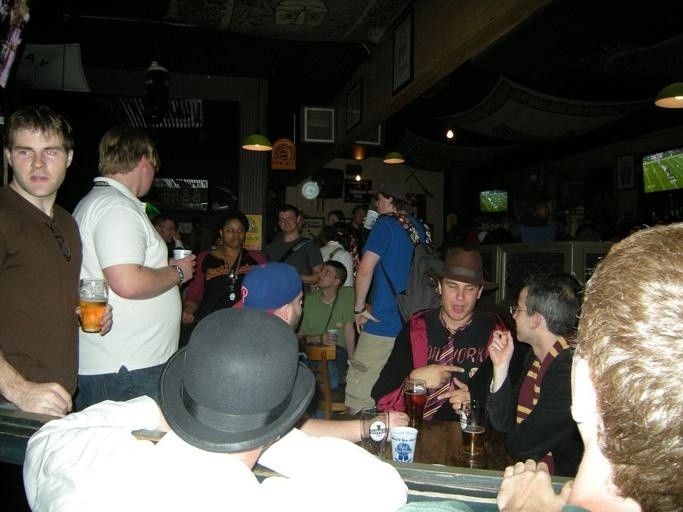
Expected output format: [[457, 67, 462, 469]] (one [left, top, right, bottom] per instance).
[[390, 5, 414, 96], [343, 77, 364, 134], [299, 106, 335, 144]]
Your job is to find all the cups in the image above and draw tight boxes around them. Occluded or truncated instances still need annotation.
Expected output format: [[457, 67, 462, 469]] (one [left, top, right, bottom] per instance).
[[359, 405, 390, 462], [327, 329, 337, 345], [459, 398, 488, 457], [387, 426, 420, 464], [364, 209, 380, 232], [77, 277, 111, 335], [173, 248, 194, 262], [402, 378, 427, 428]]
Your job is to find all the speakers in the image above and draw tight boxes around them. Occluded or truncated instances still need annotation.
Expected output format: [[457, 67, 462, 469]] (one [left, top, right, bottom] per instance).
[[319, 168, 344, 198]]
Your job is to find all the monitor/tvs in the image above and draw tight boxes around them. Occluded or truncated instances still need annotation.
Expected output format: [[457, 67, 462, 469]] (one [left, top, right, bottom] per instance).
[[476, 188, 510, 215], [638, 146, 682, 196]]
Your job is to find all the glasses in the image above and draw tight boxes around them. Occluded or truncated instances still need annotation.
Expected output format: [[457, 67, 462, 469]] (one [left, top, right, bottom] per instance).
[[506, 304, 537, 316]]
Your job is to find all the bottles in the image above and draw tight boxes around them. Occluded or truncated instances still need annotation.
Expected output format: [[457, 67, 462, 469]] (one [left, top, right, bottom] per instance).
[[227, 265, 239, 303]]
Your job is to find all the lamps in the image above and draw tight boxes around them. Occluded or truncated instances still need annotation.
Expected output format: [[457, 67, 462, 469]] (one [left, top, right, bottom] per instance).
[[238, 21, 272, 152], [383, 148, 405, 168], [652, 79, 682, 111]]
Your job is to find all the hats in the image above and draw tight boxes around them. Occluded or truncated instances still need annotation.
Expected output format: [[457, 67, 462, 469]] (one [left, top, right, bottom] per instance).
[[367, 182, 414, 205], [231, 261, 302, 311], [427, 244, 499, 292], [158, 306, 319, 452]]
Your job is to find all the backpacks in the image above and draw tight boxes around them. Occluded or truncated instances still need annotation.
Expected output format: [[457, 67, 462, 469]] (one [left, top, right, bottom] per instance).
[[376, 214, 441, 324]]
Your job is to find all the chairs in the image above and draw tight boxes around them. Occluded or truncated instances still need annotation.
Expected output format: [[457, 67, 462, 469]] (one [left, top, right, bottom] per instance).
[[299, 344, 350, 423]]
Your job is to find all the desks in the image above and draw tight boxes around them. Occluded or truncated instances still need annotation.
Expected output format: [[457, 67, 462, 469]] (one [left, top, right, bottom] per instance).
[[327, 412, 513, 471]]
